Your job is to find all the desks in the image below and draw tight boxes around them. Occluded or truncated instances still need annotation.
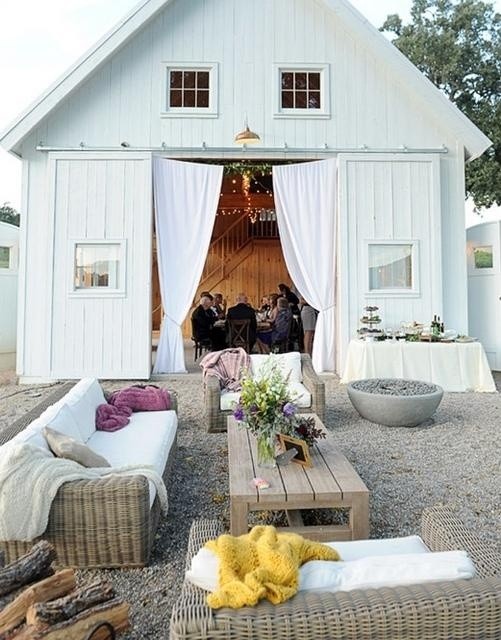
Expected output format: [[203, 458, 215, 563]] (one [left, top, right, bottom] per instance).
[[340, 337, 497, 393]]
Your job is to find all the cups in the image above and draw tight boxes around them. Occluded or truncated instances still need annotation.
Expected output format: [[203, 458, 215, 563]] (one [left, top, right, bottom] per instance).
[[399, 329, 405, 342], [385, 327, 393, 341]]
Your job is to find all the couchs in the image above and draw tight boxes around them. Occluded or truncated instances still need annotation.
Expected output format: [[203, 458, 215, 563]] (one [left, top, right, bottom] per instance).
[[0, 380, 179, 571]]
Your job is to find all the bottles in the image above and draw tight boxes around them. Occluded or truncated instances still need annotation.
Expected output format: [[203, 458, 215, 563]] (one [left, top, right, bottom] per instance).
[[431, 314, 444, 334]]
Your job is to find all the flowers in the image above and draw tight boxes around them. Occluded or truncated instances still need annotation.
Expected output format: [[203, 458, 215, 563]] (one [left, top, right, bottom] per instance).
[[227, 348, 327, 462]]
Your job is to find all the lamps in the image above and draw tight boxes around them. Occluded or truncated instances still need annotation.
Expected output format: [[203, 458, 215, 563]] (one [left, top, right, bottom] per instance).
[[234, 111, 261, 148]]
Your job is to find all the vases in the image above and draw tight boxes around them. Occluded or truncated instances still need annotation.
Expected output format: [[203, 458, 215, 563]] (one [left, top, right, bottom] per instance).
[[257, 429, 277, 469]]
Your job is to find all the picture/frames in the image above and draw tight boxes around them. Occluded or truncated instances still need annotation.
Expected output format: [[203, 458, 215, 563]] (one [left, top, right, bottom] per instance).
[[276, 432, 310, 467]]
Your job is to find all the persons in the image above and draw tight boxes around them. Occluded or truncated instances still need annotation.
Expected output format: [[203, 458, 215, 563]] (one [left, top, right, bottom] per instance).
[[191, 284, 316, 354]]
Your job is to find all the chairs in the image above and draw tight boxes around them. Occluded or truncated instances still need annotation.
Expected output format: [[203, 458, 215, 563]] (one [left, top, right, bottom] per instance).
[[203, 352, 325, 433], [191, 317, 211, 362], [168, 504, 501, 640], [227, 318, 250, 353]]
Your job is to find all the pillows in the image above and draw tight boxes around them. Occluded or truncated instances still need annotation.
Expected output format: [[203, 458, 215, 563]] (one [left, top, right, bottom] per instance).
[[42, 425, 113, 467]]
